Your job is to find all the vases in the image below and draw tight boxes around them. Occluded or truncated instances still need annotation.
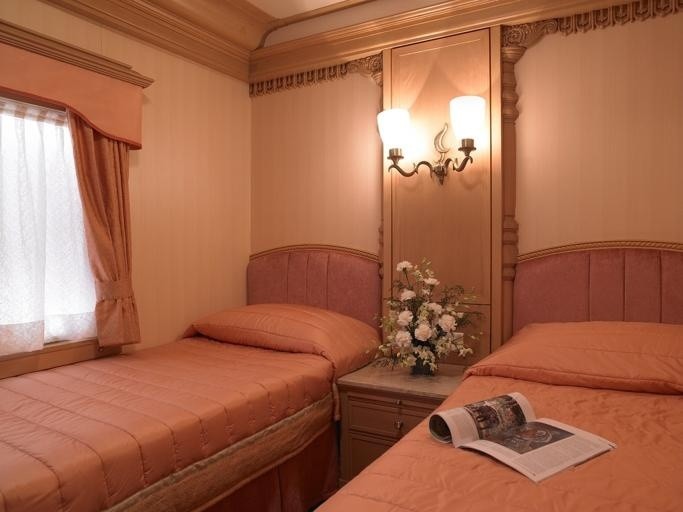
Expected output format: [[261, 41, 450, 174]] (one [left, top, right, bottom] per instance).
[[408, 351, 435, 376]]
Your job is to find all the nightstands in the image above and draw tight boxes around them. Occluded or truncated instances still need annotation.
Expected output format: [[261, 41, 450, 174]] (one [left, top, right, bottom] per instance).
[[331, 357, 470, 489]]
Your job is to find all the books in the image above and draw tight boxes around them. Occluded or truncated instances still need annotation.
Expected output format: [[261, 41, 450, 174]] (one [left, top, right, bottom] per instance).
[[429, 392, 617, 485]]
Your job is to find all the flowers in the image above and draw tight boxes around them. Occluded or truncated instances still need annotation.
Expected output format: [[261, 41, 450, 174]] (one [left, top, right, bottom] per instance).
[[364, 258, 488, 373]]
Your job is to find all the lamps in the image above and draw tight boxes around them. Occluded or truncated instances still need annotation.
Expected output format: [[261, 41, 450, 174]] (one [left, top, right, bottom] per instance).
[[374, 93, 487, 187]]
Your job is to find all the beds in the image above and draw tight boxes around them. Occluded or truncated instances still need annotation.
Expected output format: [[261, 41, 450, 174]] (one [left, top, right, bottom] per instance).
[[3, 243, 378, 510], [312, 237, 682, 512]]
